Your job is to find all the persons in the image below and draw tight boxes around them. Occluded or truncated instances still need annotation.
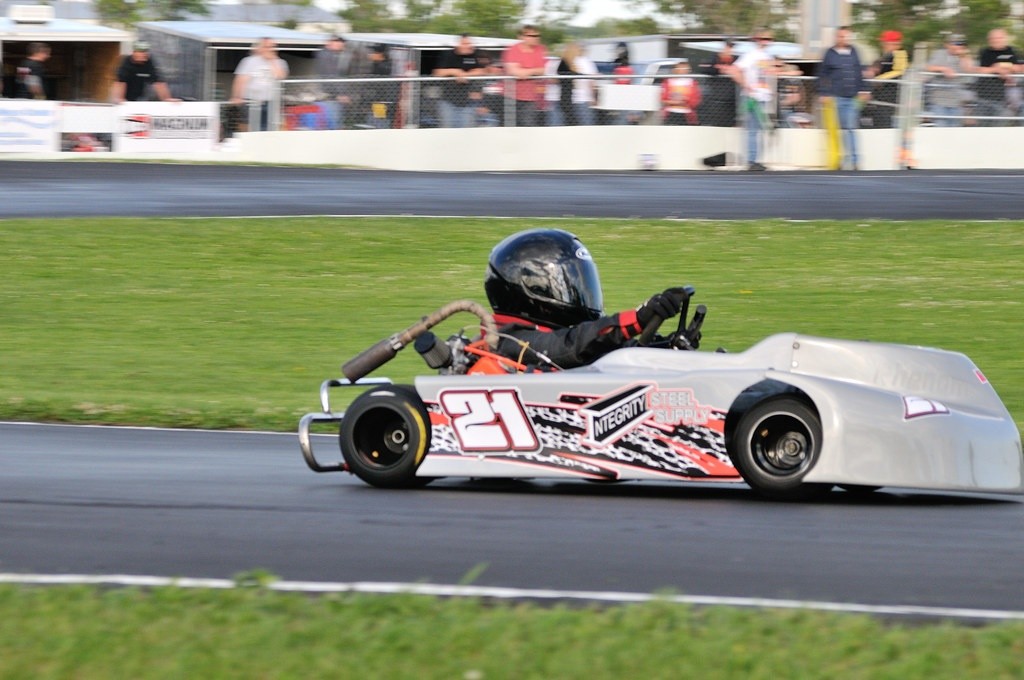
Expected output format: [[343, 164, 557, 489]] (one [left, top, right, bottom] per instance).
[[660, 33, 806, 170], [110, 44, 182, 102], [924, 28, 1024, 127], [16, 44, 50, 99], [612, 43, 629, 67], [229, 38, 288, 130], [432, 24, 598, 126], [314, 35, 390, 128], [483, 227, 702, 370], [819, 28, 907, 168]]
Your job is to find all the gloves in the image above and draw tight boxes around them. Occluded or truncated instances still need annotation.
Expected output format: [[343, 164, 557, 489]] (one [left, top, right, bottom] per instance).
[[665, 321, 702, 349], [638, 287, 684, 327]]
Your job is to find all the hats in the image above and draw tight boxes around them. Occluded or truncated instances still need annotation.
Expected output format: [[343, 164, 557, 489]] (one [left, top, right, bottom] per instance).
[[134, 40, 153, 56], [880, 31, 902, 47]]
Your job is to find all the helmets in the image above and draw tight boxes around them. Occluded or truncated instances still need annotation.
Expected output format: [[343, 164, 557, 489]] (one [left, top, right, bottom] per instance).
[[485, 224, 602, 328]]
